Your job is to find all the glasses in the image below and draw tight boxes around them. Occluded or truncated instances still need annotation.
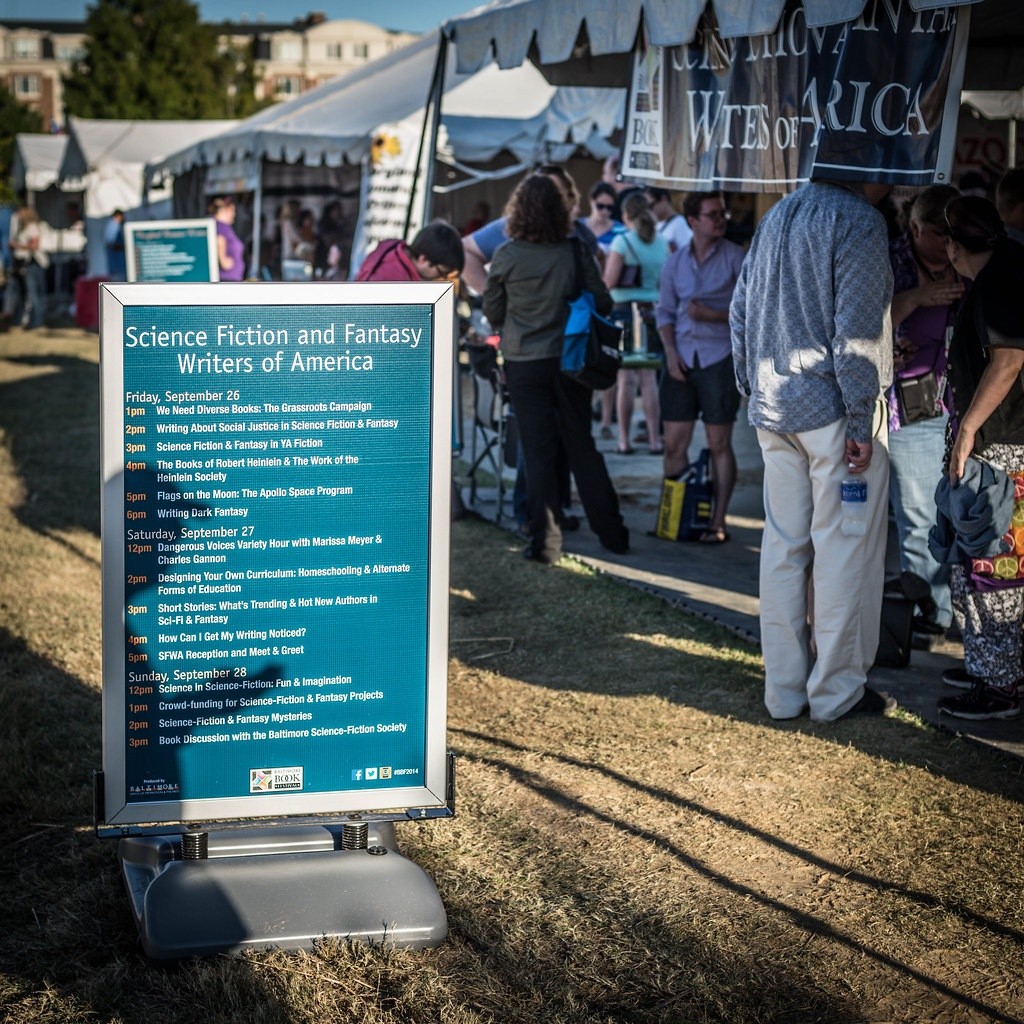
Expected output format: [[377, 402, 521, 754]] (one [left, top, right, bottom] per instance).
[[943, 198, 957, 234], [433, 262, 449, 280], [688, 211, 734, 221], [592, 198, 615, 211]]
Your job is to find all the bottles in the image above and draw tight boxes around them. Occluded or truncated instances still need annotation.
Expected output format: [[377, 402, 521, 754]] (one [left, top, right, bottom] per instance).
[[841, 463, 869, 537]]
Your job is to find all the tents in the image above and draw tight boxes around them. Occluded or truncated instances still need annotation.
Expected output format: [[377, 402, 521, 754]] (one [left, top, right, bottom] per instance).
[[11, 0, 1023, 276]]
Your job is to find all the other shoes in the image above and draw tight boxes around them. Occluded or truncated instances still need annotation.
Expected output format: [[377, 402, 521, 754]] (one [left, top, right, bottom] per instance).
[[633, 433, 649, 442], [841, 690, 897, 717], [707, 522, 727, 543], [912, 614, 948, 650]]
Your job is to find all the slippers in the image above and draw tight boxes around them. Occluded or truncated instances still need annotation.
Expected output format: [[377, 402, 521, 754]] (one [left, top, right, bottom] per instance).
[[616, 444, 634, 454], [649, 441, 663, 454]]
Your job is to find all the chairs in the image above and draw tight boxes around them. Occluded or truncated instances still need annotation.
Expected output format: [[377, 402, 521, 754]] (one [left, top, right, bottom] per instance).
[[459, 343, 518, 527]]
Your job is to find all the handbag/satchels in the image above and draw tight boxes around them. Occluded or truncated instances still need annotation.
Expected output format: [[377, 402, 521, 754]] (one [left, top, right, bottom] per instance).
[[895, 375, 942, 425], [872, 591, 913, 666], [560, 293, 622, 391], [612, 236, 642, 289], [657, 454, 715, 542]]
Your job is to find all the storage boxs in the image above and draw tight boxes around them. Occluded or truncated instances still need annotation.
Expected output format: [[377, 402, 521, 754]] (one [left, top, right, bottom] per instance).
[[874, 572, 916, 670], [73, 275, 108, 330]]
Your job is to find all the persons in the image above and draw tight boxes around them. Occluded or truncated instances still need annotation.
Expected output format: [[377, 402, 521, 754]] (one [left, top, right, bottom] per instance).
[[727, 168, 1023, 722], [579, 154, 693, 455], [87, 210, 124, 278], [207, 196, 244, 282], [1, 202, 81, 330], [245, 199, 356, 281], [646, 192, 745, 544], [354, 223, 465, 282], [459, 165, 630, 563]]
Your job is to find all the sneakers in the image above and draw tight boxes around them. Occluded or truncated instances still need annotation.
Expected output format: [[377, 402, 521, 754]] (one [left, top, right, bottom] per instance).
[[942, 667, 1024, 690], [938, 679, 1019, 720]]
